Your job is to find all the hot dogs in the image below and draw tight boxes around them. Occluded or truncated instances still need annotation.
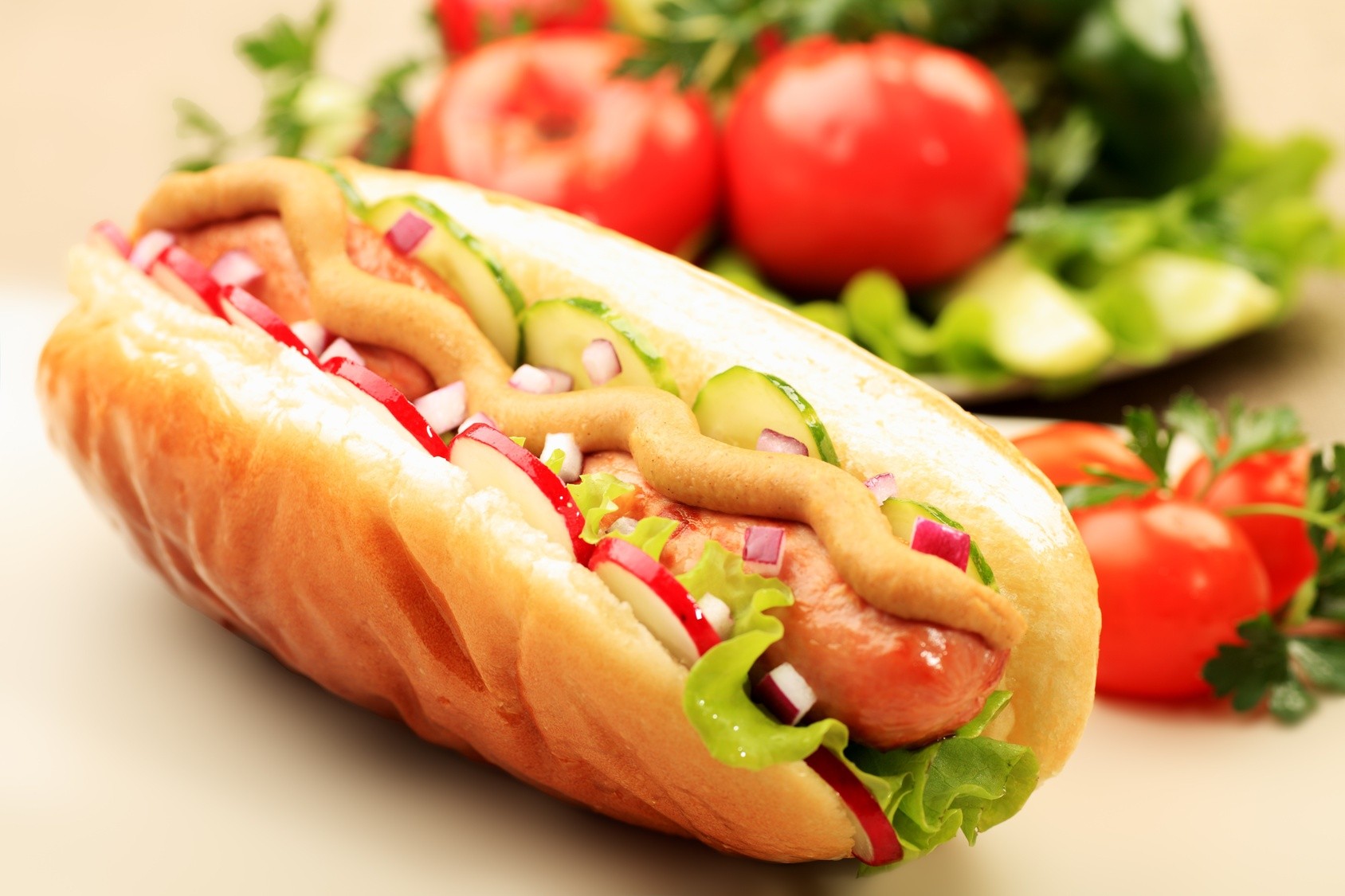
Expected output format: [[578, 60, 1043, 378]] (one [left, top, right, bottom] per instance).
[[35, 155, 1106, 870]]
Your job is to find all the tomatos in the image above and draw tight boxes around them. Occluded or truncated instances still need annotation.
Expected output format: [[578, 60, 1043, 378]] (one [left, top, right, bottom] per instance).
[[990, 420, 1335, 703], [398, 24, 1026, 289]]
